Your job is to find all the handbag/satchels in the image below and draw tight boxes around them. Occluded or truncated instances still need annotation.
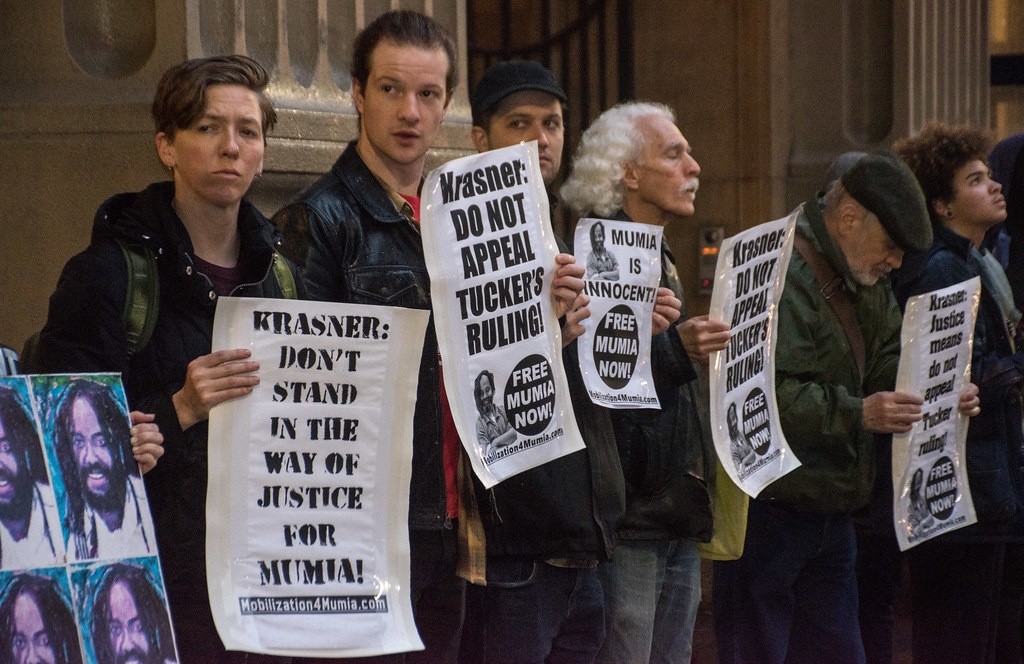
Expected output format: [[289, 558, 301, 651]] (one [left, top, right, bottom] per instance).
[[691, 457, 749, 562]]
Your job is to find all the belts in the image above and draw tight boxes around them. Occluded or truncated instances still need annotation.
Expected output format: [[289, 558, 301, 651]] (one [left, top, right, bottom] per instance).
[[545, 558, 599, 568]]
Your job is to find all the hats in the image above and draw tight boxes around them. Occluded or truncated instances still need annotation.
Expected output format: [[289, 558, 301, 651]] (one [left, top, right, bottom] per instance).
[[842, 150, 934, 252], [470, 59, 567, 110]]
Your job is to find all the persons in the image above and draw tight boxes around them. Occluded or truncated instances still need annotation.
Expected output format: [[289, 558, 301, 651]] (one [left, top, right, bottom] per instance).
[[559, 100, 728, 664], [0, 342, 168, 664], [271, 9, 585, 664], [727, 402, 756, 471], [907, 467, 934, 536], [585, 221, 620, 282], [828, 128, 1024, 663], [473, 370, 517, 454], [8, 52, 314, 664], [697, 145, 984, 664], [467, 53, 681, 664]]
